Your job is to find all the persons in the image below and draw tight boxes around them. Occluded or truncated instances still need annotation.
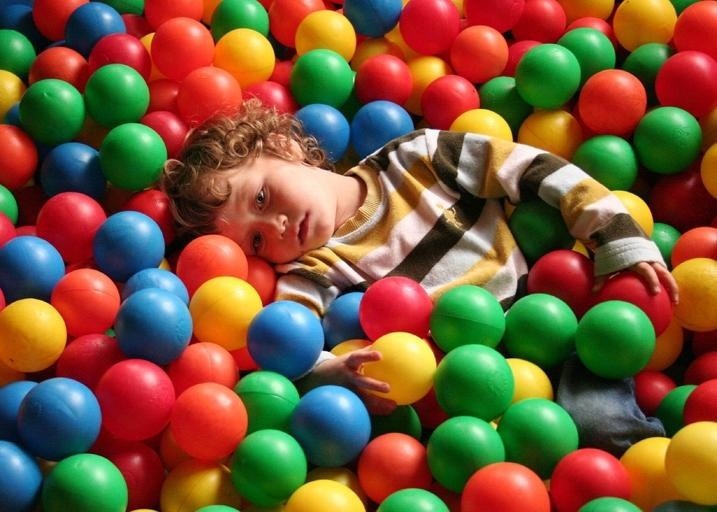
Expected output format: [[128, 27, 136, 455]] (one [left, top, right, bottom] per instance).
[[160, 97, 679, 460]]
